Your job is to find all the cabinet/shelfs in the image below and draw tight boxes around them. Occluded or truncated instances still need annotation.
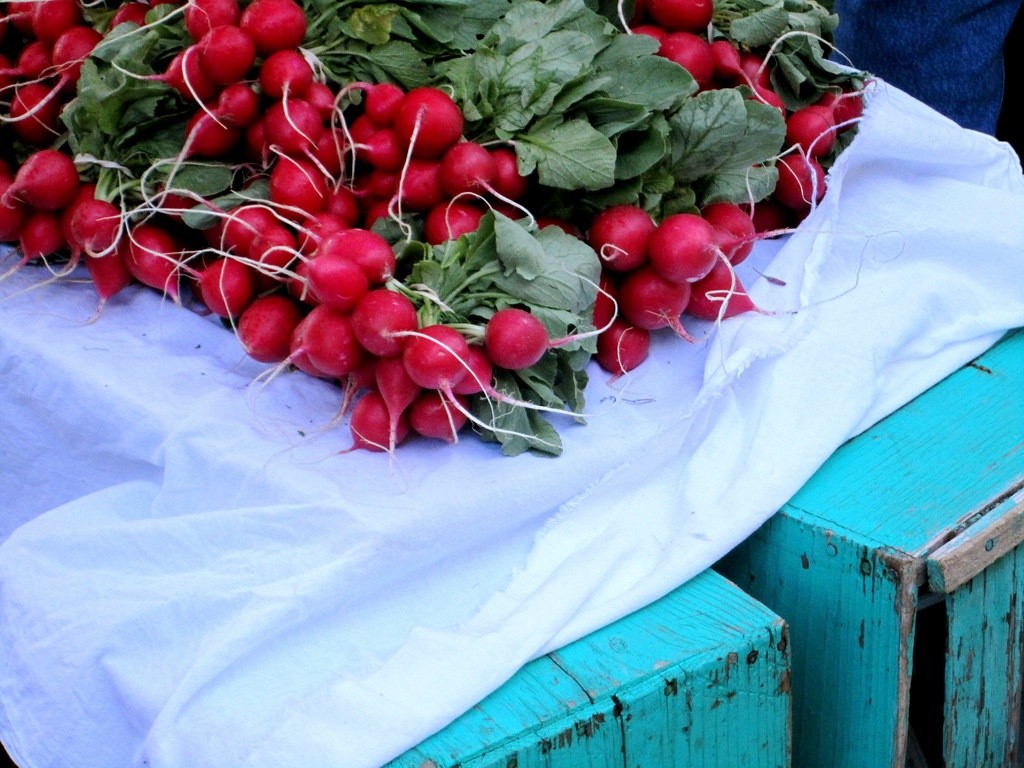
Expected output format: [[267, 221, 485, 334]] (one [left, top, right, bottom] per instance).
[[383, 328, 1024, 768]]
